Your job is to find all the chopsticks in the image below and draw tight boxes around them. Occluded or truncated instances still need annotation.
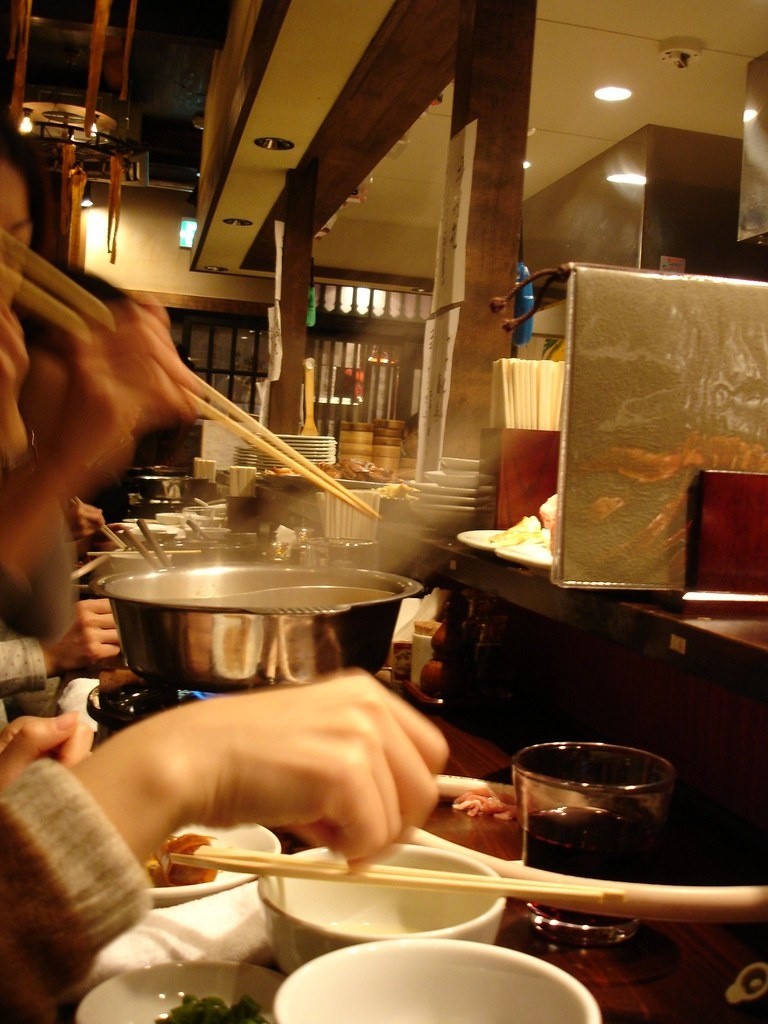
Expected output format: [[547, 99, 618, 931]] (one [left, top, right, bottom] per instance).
[[487, 357, 567, 431], [192, 458, 216, 485], [323, 488, 381, 543], [67, 495, 125, 552], [0, 227, 385, 525], [125, 520, 169, 568], [169, 840, 627, 905], [227, 468, 258, 497]]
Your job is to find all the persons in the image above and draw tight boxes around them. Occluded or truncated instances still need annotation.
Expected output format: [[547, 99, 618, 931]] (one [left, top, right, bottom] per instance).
[[401, 413, 419, 458], [0, 112, 452, 1024]]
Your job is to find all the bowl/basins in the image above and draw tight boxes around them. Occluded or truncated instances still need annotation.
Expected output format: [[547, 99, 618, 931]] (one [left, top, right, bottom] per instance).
[[257, 841, 508, 975], [272, 938, 602, 1024], [76, 961, 290, 1024]]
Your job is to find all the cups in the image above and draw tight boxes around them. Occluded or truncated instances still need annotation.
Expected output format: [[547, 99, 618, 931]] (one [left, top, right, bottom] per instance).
[[411, 620, 445, 686], [511, 742, 678, 944], [475, 428, 563, 532], [227, 495, 258, 526]]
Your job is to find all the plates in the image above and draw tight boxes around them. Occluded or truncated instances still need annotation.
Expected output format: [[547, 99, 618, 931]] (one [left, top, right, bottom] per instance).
[[269, 472, 396, 492], [113, 503, 229, 550], [145, 823, 281, 908], [455, 528, 558, 569], [407, 457, 495, 535], [232, 432, 336, 471]]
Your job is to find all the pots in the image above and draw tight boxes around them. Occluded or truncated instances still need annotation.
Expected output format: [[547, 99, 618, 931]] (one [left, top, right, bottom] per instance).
[[117, 467, 214, 502], [91, 564, 424, 698]]
